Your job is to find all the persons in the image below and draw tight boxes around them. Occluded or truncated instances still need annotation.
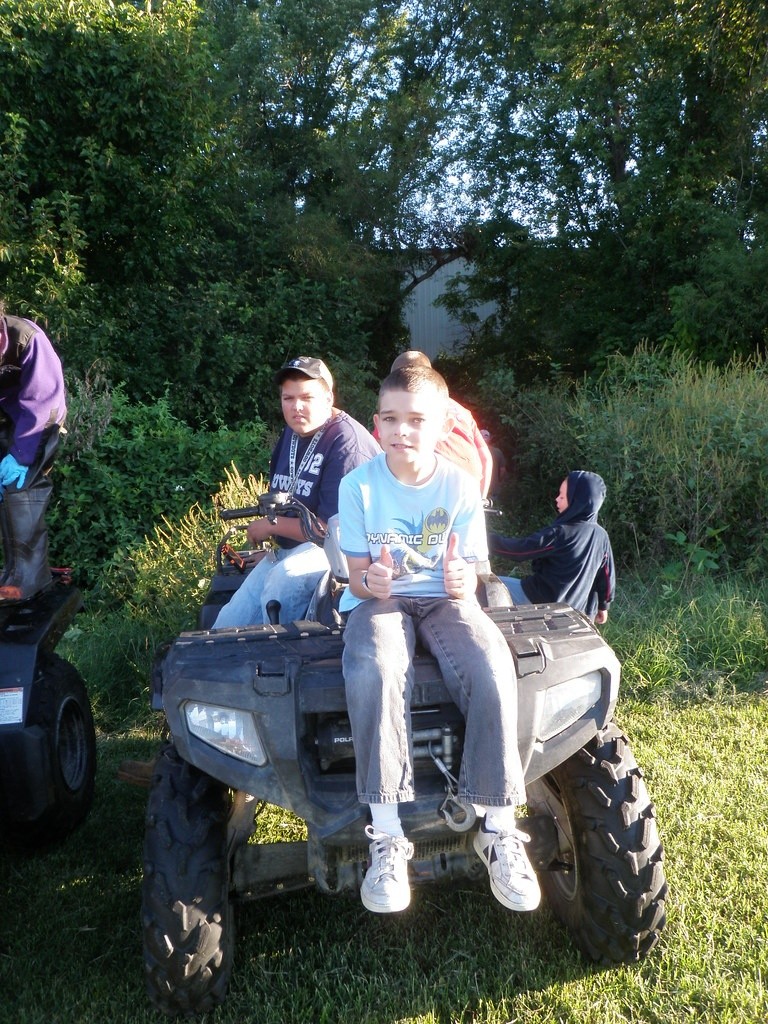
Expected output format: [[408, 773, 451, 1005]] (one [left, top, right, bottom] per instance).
[[211, 356, 384, 629], [338, 365, 542, 914], [372, 349, 493, 501], [487, 471, 617, 624], [480, 429, 506, 500], [0, 300, 68, 600]]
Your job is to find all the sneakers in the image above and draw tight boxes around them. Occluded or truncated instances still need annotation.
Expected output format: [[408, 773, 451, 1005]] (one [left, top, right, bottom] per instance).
[[473, 813, 542, 912], [360, 825, 414, 912]]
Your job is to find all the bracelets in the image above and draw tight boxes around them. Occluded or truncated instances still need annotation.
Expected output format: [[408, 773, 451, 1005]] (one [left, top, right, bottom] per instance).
[[362, 573, 372, 594]]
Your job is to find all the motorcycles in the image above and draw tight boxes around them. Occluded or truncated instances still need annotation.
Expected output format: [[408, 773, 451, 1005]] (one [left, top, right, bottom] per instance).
[[0, 568, 97, 853], [139, 485, 672, 1013]]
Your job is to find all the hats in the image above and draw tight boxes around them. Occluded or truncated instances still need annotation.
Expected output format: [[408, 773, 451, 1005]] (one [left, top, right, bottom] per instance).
[[275, 356, 334, 392]]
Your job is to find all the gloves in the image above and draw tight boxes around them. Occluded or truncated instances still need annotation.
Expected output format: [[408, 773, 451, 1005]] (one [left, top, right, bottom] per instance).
[[0, 454, 29, 489]]
